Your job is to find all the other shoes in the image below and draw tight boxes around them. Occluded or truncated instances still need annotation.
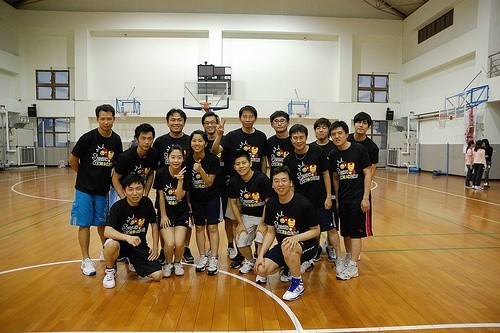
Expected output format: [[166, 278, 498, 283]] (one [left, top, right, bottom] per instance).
[[465, 182, 489, 190]]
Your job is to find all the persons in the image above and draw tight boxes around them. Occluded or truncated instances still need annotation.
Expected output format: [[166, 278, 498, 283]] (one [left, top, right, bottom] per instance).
[[327, 121, 371, 279], [152, 146, 191, 278], [67, 104, 124, 275], [263, 109, 296, 181], [465, 139, 493, 190], [101, 173, 164, 287], [211, 105, 269, 270], [153, 108, 196, 262], [254, 166, 320, 301], [227, 150, 272, 283], [280, 123, 346, 275], [347, 112, 379, 260], [308, 118, 341, 262], [184, 129, 221, 276], [109, 123, 157, 209], [199, 112, 239, 260]]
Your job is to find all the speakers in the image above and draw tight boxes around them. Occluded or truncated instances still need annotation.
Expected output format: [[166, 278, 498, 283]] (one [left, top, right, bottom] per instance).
[[386, 111, 394, 120], [28, 107, 36, 117]]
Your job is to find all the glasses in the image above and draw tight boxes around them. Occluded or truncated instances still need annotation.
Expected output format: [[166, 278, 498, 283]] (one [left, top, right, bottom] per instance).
[[272, 119, 286, 123]]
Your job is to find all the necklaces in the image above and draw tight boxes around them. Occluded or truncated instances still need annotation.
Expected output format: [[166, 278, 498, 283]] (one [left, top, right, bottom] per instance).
[[296, 152, 306, 160]]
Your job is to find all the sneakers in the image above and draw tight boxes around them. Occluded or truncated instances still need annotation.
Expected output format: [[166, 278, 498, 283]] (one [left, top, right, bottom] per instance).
[[256, 274, 267, 283], [196, 254, 207, 272], [334, 258, 345, 274], [173, 263, 185, 276], [325, 246, 336, 261], [162, 261, 173, 277], [336, 262, 359, 280], [81, 258, 96, 276], [100, 251, 105, 261], [239, 259, 254, 274], [300, 260, 314, 274], [205, 248, 211, 256], [230, 255, 245, 269], [227, 248, 236, 259], [157, 248, 165, 265], [343, 253, 351, 265], [282, 277, 305, 301], [102, 268, 117, 289], [127, 259, 136, 272], [313, 245, 322, 261], [207, 256, 218, 275], [182, 246, 195, 263], [279, 265, 293, 282]]
